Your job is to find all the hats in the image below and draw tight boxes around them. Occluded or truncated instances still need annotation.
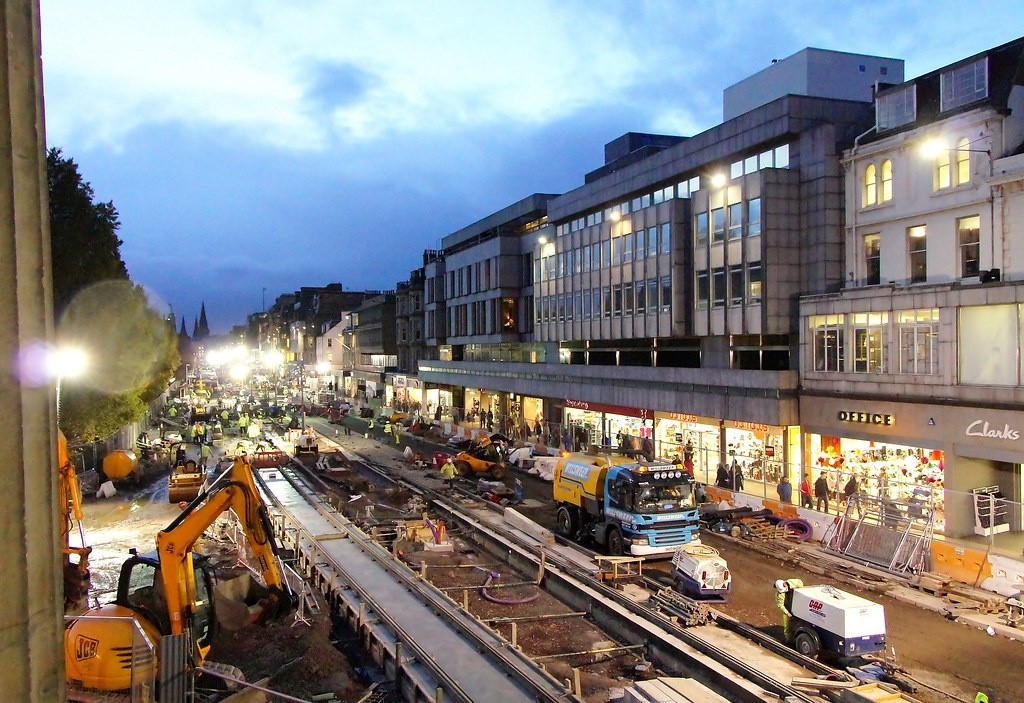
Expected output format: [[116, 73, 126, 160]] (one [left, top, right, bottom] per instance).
[[775, 579, 787, 592], [447, 458, 452, 463], [386, 421, 390, 423]]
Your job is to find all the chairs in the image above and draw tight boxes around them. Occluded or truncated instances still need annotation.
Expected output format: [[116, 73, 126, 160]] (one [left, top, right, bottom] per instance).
[[187, 460, 196, 472]]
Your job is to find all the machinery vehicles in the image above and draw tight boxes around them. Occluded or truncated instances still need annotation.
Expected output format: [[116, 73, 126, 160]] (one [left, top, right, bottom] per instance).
[[64, 448, 300, 703], [168, 441, 208, 504], [454, 432, 513, 477], [56, 426, 93, 612]]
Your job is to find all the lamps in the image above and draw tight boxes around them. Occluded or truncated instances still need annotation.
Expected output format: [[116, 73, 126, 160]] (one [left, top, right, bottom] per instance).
[[928, 417, 935, 426]]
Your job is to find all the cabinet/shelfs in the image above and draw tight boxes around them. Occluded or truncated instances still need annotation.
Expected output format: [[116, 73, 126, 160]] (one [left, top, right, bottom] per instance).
[[973, 485, 998, 536], [987, 486, 1011, 533]]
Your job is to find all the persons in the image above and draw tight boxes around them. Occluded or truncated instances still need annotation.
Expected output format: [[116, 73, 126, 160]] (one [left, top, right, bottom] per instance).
[[713, 459, 745, 492], [673, 440, 695, 478], [776, 468, 904, 536], [163, 380, 394, 471], [396, 396, 655, 470], [775, 578, 803, 645], [441, 459, 459, 492]]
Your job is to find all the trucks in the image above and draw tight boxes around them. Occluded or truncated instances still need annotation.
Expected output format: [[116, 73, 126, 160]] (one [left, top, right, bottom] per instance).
[[553, 442, 705, 561]]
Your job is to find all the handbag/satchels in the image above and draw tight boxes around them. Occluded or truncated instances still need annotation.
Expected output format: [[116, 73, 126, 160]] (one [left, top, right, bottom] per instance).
[[714, 478, 718, 486], [725, 478, 731, 488]]
